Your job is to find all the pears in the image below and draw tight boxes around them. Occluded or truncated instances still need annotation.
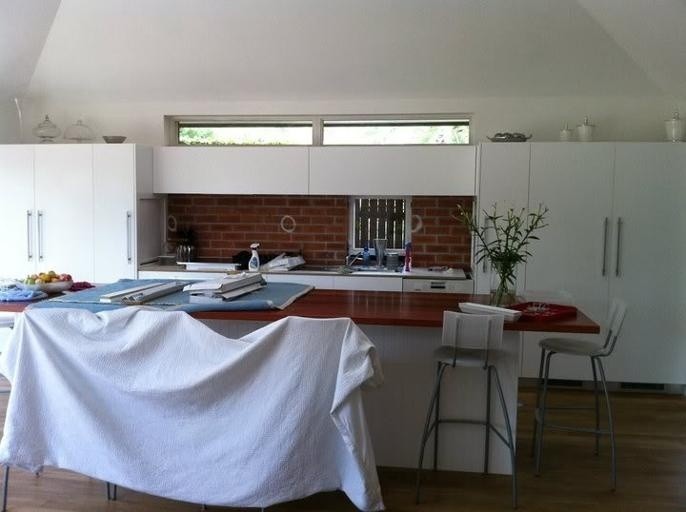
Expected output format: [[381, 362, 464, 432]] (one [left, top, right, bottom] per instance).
[[26, 277, 35, 285], [24, 274, 31, 284]]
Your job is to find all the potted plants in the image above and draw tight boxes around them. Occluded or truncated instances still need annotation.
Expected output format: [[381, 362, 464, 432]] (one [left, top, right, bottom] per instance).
[[449, 200, 550, 308]]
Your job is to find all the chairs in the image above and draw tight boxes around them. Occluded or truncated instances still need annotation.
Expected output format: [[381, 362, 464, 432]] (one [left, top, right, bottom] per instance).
[[413, 308, 522, 511], [1, 460, 114, 512], [197, 502, 271, 512], [531, 295, 630, 491]]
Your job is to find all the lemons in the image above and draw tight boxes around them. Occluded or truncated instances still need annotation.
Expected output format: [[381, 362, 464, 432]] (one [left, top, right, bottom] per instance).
[[40, 273, 50, 283]]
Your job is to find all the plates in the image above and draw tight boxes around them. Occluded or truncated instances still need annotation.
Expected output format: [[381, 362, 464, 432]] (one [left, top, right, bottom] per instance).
[[16, 279, 73, 293], [458, 301, 523, 324], [102, 136, 126, 144]]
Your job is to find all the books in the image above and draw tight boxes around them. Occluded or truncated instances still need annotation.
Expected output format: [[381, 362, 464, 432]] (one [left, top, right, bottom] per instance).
[[181, 270, 269, 305]]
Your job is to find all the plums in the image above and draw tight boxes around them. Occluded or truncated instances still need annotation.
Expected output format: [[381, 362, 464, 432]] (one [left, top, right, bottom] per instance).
[[60, 276, 65, 281], [64, 274, 72, 281]]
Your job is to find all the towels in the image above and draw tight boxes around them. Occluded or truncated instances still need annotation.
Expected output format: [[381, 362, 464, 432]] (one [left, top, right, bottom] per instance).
[[0, 287, 48, 301]]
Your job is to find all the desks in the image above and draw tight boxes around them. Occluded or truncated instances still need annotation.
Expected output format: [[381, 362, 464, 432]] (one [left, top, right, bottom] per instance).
[[0, 283, 601, 512]]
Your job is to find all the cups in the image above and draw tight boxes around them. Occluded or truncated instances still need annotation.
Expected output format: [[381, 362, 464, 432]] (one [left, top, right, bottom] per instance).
[[665, 113, 685, 142], [560, 123, 573, 142], [373, 238, 388, 268], [577, 119, 594, 142]]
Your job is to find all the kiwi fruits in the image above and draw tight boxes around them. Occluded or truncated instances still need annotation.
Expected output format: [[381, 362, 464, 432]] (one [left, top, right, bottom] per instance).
[[48, 271, 55, 276], [31, 273, 38, 280], [51, 277, 62, 283]]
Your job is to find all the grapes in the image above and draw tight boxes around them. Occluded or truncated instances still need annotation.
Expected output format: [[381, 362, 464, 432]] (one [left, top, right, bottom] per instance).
[[70, 281, 92, 290]]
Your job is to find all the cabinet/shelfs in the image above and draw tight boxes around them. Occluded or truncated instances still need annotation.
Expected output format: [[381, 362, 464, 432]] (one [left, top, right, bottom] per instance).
[[0, 144, 93, 282], [93, 143, 162, 284], [310, 145, 477, 196], [527, 140, 686, 393], [153, 146, 310, 194], [470, 142, 529, 297]]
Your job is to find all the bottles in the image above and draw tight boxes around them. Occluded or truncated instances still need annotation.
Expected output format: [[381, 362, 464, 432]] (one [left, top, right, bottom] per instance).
[[362, 246, 370, 268], [387, 252, 399, 270], [404, 242, 413, 271]]
